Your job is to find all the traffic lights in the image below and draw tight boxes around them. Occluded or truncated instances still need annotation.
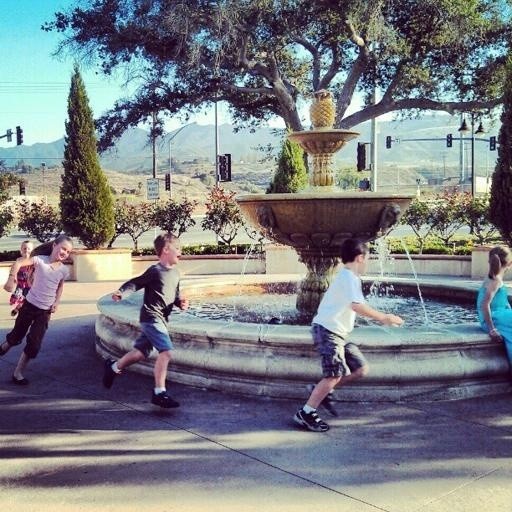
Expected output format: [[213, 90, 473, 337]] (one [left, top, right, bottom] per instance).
[[446, 134, 452, 147], [17, 126, 23, 145], [490, 136, 495, 151], [386, 136, 391, 148]]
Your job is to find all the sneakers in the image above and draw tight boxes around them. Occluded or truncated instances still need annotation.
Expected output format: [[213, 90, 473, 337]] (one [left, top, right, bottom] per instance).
[[103, 358, 118, 389], [311, 383, 338, 417], [152, 387, 181, 407], [0, 341, 10, 355], [13, 370, 28, 384], [11, 307, 19, 316], [293, 407, 329, 432]]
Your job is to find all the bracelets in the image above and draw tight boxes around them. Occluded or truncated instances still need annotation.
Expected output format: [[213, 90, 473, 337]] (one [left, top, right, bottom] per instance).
[[9, 273, 16, 281]]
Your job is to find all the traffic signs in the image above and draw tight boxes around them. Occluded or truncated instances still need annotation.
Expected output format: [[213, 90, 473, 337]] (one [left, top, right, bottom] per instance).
[[394, 138, 400, 143]]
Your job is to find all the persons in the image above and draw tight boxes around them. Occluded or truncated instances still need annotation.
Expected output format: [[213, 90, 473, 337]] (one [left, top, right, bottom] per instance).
[[8, 239, 35, 318], [102, 233, 190, 408], [475, 244, 512, 368], [292, 237, 405, 433], [0, 233, 75, 387]]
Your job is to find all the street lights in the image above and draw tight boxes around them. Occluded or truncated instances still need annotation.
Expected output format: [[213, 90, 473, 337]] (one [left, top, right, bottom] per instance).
[[166, 122, 197, 234], [457, 108, 487, 235], [41, 161, 46, 201]]
